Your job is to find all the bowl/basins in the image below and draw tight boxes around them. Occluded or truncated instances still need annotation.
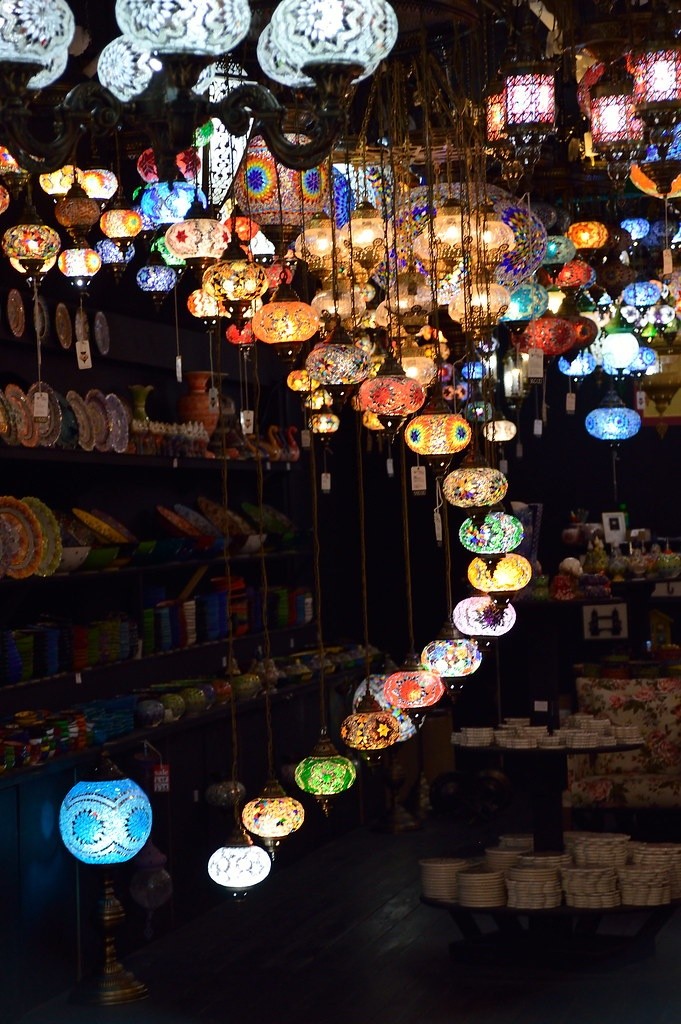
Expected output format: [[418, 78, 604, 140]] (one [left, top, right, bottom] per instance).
[[80, 548, 120, 570], [53, 546, 91, 576], [137, 534, 267, 562]]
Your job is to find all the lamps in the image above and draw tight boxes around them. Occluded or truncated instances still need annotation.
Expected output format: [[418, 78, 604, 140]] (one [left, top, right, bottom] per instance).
[[0, 0, 680, 897]]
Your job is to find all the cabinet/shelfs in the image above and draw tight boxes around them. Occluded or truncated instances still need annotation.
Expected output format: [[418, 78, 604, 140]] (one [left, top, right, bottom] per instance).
[[1, 296, 390, 1022]]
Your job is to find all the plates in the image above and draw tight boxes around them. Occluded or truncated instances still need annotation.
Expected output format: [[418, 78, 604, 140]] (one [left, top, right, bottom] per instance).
[[0, 518, 12, 580], [418, 831, 681, 909], [52, 508, 138, 547], [157, 496, 296, 537], [7, 289, 110, 355], [11, 576, 314, 683], [450, 716, 645, 749], [0, 381, 129, 454], [0, 496, 43, 580], [20, 496, 63, 576]]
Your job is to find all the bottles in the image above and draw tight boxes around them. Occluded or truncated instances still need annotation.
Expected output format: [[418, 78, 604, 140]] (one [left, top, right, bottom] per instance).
[[179, 371, 235, 444]]
